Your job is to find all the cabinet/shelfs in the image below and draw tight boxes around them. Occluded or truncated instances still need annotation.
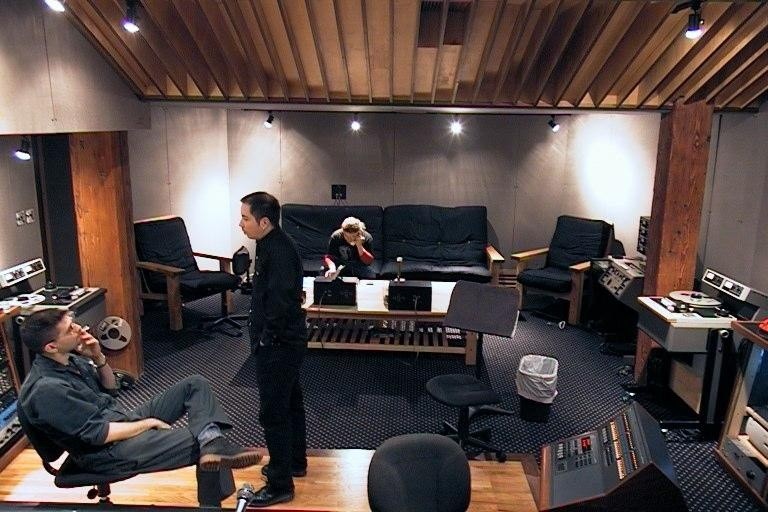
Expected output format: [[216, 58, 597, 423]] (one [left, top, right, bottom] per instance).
[[713, 321, 767, 511]]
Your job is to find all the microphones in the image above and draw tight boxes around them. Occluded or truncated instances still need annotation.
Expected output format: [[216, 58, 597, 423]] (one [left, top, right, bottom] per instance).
[[234, 483, 255, 512]]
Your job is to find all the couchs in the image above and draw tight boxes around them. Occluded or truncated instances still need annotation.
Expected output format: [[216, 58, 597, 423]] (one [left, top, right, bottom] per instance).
[[280, 204, 505, 284]]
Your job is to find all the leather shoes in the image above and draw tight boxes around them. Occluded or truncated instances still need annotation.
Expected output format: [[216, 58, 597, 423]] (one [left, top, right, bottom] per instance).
[[248, 486, 294, 507], [261, 464, 306, 477]]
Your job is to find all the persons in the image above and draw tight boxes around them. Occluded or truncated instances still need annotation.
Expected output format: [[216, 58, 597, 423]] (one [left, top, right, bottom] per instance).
[[324, 217, 374, 280], [239, 191, 307, 508], [21, 308, 262, 506]]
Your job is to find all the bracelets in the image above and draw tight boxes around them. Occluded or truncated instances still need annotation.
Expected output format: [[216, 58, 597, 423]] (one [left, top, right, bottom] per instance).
[[90, 352, 108, 369]]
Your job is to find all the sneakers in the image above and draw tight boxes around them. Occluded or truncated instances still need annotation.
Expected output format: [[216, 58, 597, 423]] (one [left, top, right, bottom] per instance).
[[199, 437, 263, 472]]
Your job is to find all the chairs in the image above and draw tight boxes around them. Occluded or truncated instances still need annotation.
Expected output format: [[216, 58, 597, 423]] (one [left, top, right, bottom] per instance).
[[367, 433, 471, 511], [425, 281, 524, 462], [17, 401, 138, 510], [131, 215, 241, 343], [510, 214, 615, 327]]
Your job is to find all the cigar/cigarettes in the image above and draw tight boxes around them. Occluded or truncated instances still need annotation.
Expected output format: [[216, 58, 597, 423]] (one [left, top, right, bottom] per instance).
[[80, 327, 90, 333]]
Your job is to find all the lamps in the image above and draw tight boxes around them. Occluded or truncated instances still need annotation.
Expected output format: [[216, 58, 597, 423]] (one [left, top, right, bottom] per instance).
[[264, 114, 274, 129], [122, 0, 140, 34], [673, 0, 703, 41], [46, 0, 66, 12], [14, 134, 31, 161], [547, 120, 559, 132], [351, 115, 360, 131]]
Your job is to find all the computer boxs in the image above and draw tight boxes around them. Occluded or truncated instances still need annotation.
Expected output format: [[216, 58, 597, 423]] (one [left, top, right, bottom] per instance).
[[313, 276, 358, 306], [388, 280, 431, 311]]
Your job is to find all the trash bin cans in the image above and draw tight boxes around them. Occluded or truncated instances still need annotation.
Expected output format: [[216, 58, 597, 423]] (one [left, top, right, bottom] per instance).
[[515, 354, 559, 424]]
